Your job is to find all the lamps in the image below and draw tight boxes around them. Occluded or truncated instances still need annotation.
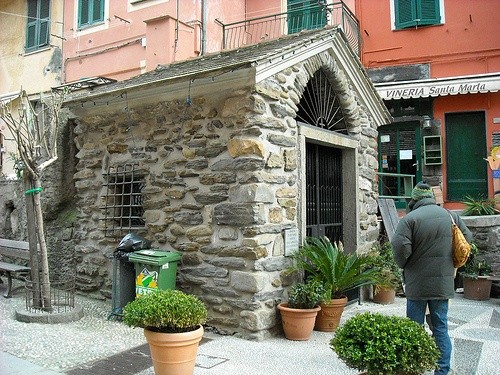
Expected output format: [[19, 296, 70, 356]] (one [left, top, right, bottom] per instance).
[[423, 116, 431, 129]]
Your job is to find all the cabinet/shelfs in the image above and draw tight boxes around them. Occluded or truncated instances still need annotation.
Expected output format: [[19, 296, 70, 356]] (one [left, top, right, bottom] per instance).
[[378, 172, 416, 203], [423, 135, 444, 166]]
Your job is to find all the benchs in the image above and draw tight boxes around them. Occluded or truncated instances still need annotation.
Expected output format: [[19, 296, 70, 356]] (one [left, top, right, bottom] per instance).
[[0, 238, 40, 298]]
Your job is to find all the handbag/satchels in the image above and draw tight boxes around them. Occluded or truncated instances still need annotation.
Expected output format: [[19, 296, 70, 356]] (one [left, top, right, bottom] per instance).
[[446, 211, 471, 268]]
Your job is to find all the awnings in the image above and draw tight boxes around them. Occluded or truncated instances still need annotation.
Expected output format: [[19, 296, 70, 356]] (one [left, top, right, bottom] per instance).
[[372, 72, 500, 100]]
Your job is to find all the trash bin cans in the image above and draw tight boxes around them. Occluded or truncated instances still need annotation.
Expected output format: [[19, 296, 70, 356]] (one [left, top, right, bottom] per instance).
[[128, 248, 183, 296], [112, 247, 136, 316]]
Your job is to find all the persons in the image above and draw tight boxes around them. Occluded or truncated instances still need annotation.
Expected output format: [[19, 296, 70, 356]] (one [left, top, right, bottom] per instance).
[[391, 181, 472, 375]]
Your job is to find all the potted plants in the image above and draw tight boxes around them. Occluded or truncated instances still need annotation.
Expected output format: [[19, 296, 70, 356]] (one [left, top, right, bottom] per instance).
[[299, 236, 380, 331], [276, 280, 326, 341], [362, 247, 395, 305], [122, 289, 208, 375], [459, 256, 492, 301]]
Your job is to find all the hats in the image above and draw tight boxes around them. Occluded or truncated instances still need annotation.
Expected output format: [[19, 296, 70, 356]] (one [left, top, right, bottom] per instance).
[[410, 180, 435, 200]]
